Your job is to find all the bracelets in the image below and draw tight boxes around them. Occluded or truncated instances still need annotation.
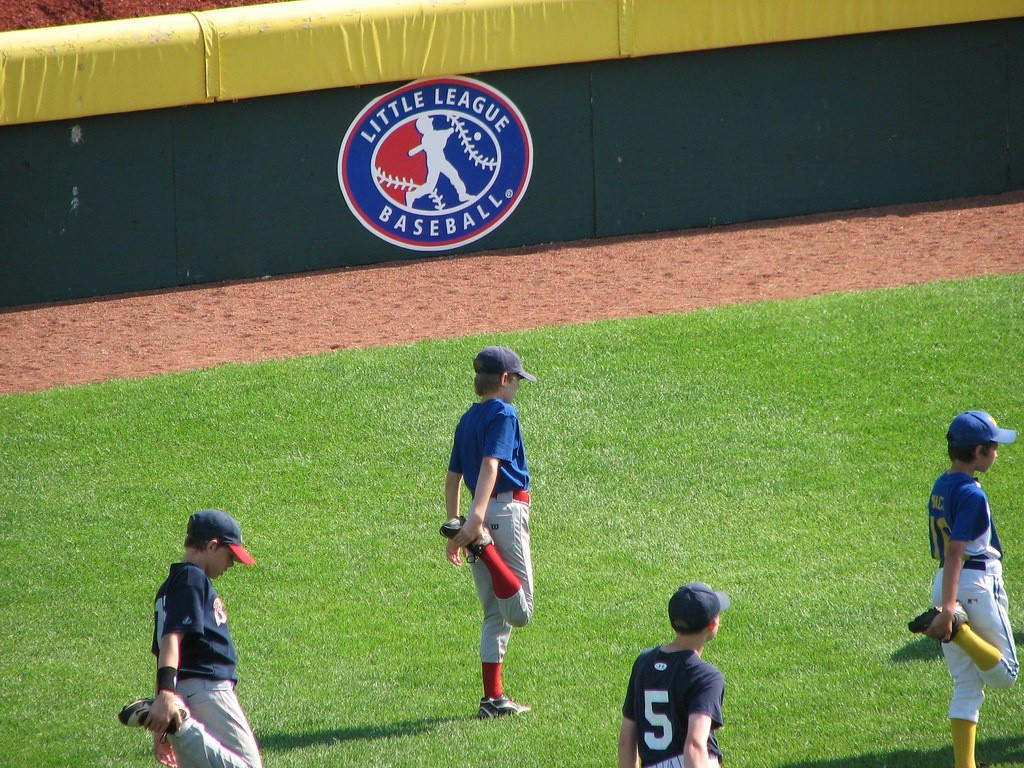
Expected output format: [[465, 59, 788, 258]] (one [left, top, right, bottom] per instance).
[[157, 665, 177, 695]]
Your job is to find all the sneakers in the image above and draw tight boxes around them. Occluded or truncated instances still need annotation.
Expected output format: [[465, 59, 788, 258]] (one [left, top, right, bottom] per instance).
[[118, 699, 192, 744], [441, 516, 495, 563], [909, 600, 968, 643], [477, 694, 532, 717]]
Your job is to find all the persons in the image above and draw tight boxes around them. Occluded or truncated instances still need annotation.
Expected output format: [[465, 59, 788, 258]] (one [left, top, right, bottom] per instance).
[[439, 345, 537, 719], [908, 411, 1020, 768], [618, 583, 730, 768], [119, 510, 263, 768]]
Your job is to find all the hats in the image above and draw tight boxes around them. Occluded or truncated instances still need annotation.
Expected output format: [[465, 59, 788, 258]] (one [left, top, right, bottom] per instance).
[[668, 582, 730, 632], [473, 347, 538, 383], [948, 411, 1016, 446], [186, 510, 256, 565]]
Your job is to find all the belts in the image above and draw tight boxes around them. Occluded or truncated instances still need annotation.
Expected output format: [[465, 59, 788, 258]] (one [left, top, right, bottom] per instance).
[[492, 488, 530, 502], [964, 561, 988, 570]]
